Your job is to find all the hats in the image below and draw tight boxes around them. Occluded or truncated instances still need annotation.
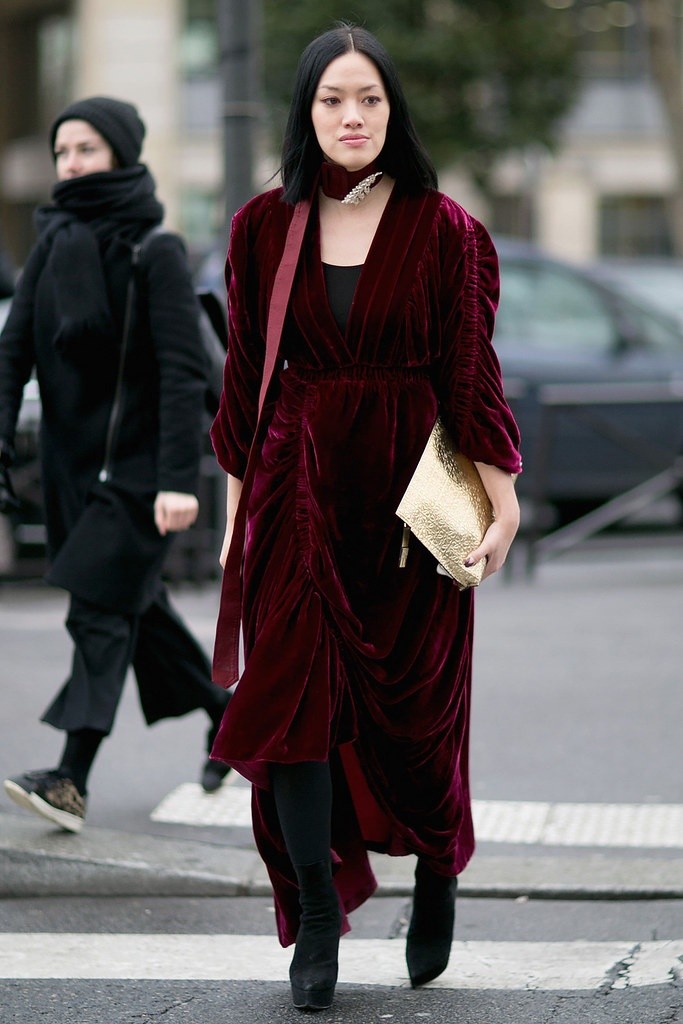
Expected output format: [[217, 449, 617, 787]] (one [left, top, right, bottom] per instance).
[[49, 97, 146, 167]]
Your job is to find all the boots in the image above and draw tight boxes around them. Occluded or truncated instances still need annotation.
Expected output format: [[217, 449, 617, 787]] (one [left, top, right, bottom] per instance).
[[271, 758, 342, 1008], [406, 856, 458, 990]]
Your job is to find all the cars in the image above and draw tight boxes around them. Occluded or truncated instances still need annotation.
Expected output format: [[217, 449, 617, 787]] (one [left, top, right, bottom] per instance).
[[1, 238, 681, 582]]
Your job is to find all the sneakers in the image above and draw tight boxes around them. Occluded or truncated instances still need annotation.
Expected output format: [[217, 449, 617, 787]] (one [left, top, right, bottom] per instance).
[[3, 770, 88, 833], [202, 731, 232, 792]]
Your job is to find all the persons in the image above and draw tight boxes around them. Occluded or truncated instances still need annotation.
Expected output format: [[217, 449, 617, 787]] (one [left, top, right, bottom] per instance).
[[211, 26, 523, 1010], [0, 96, 232, 834]]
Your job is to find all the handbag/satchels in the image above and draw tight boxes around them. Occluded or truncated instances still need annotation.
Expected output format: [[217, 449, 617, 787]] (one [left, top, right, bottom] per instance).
[[394, 414, 523, 592]]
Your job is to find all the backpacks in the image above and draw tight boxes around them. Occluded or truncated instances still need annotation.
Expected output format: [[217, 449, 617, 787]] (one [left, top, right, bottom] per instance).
[[40, 214, 229, 459]]
